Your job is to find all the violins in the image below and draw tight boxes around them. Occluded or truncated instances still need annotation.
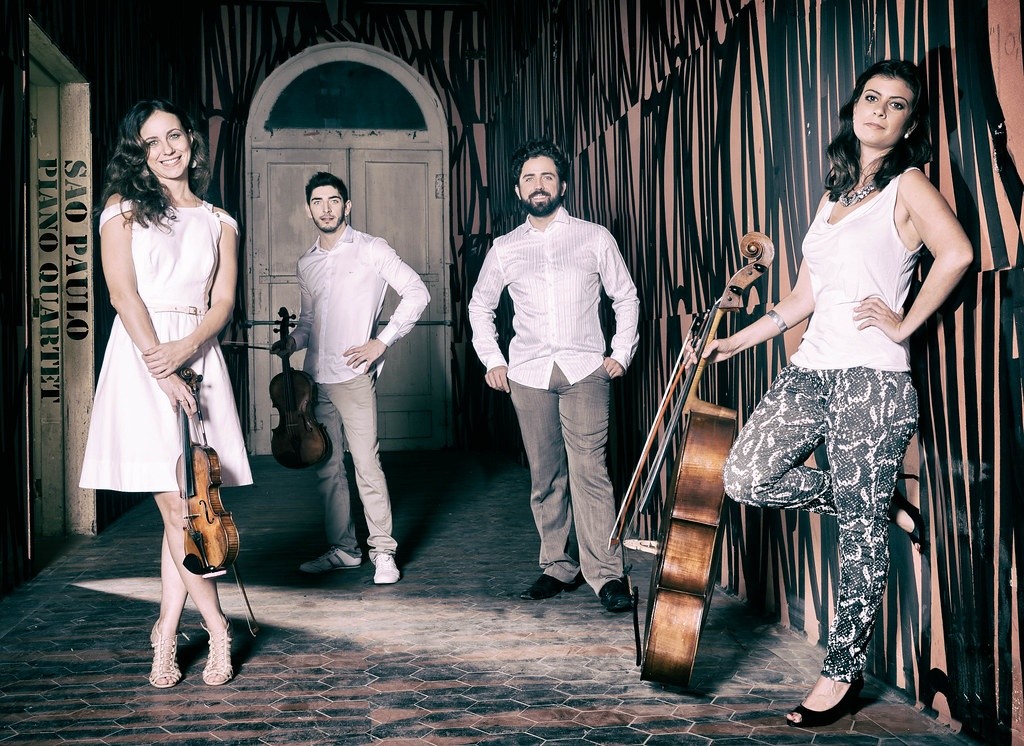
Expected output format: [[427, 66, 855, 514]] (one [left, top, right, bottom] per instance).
[[173, 365, 242, 581], [268, 305, 335, 472]]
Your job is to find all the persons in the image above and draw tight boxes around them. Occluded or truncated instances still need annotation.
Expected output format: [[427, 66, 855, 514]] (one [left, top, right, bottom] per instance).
[[269, 172, 431, 584], [78, 99, 253, 689], [468, 140, 640, 611], [684, 60, 974, 728]]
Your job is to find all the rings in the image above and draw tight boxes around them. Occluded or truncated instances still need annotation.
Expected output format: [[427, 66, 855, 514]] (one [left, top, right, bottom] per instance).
[[178, 399, 186, 402]]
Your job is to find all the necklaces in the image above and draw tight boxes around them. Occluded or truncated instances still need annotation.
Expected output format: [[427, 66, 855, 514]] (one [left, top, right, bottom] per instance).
[[838, 182, 877, 207]]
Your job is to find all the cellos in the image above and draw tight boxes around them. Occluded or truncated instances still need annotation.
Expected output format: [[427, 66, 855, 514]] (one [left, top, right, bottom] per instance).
[[631, 228, 785, 691]]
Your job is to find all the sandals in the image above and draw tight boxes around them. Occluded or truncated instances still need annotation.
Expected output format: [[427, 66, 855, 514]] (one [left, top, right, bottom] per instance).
[[149, 618, 182, 688], [200, 617, 232, 686]]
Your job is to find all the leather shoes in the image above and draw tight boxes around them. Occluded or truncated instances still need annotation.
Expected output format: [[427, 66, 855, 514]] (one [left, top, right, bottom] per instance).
[[598, 577, 632, 612], [520, 574, 576, 600]]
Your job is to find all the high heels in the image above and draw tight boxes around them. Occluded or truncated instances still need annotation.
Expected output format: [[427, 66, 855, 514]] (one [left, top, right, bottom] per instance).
[[888, 472, 926, 555], [786, 676, 865, 727]]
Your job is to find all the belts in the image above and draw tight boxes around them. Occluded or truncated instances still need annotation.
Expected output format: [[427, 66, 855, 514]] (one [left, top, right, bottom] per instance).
[[144, 305, 206, 315]]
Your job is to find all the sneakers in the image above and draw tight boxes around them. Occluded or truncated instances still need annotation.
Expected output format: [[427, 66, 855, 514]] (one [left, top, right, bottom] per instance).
[[373, 552, 400, 584], [300, 546, 361, 572]]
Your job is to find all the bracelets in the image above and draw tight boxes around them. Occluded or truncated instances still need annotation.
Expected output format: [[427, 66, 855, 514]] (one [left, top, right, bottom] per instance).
[[767, 309, 788, 333]]
